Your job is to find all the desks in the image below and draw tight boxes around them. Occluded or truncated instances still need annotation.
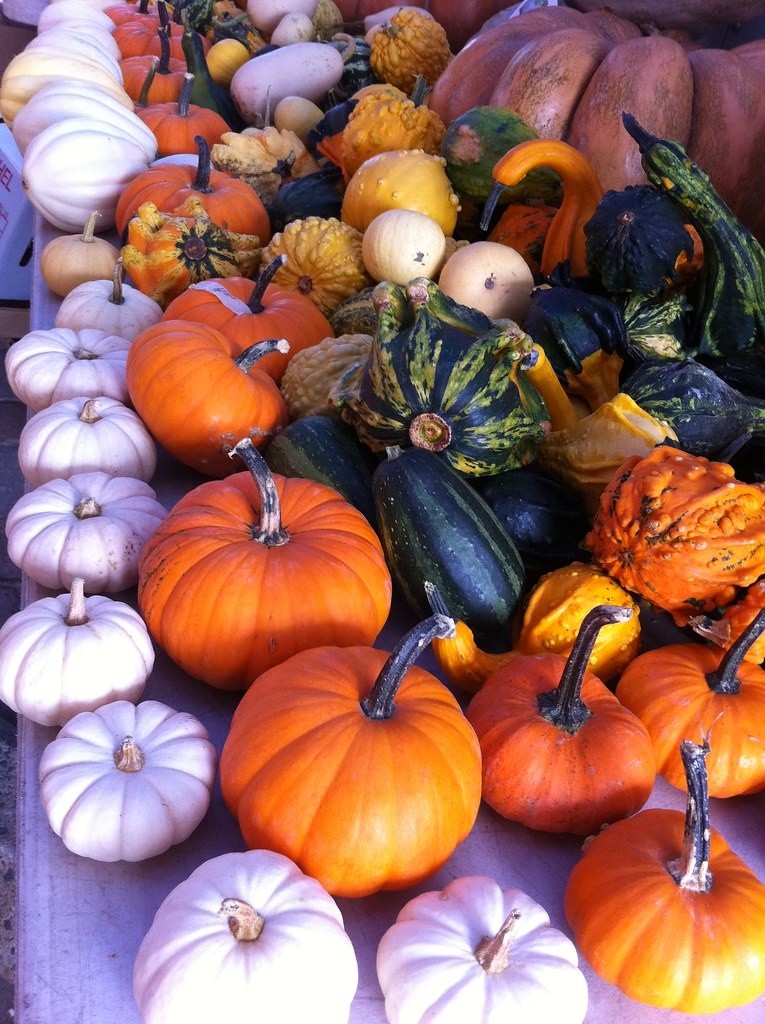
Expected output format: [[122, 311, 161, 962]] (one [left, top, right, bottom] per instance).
[[13, 214, 765, 1024]]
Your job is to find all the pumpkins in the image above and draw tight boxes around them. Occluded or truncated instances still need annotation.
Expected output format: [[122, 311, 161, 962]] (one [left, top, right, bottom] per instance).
[[0, 0, 765, 1023]]
